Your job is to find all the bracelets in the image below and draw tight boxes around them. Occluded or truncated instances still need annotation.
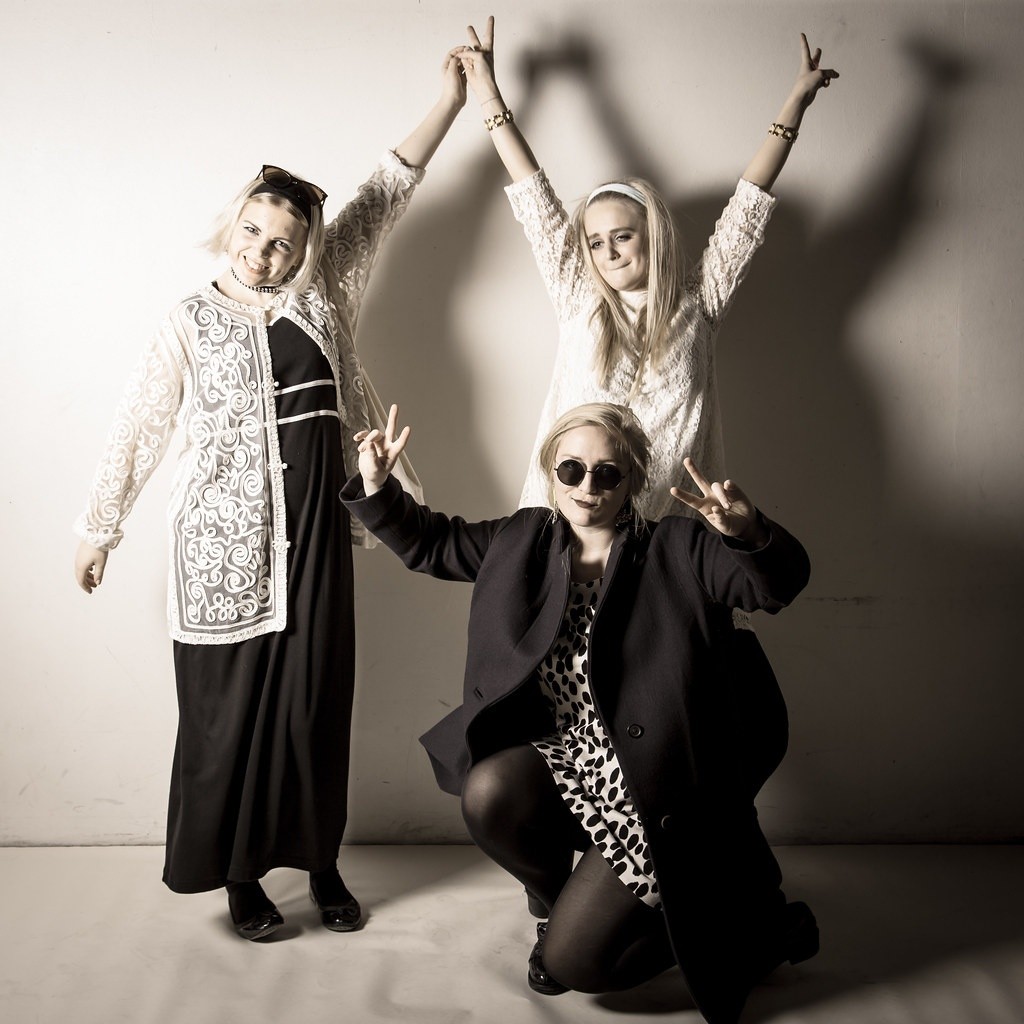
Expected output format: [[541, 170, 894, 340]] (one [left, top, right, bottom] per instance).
[[768, 123, 799, 142], [484, 110, 514, 130], [480, 95, 501, 106]]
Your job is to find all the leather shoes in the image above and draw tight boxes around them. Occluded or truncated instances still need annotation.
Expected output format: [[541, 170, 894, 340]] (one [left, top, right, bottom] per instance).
[[307, 878, 362, 934], [221, 888, 285, 942], [528, 920, 574, 996]]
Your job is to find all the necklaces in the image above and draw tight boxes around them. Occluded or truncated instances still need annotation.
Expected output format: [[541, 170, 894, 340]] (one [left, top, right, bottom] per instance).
[[230, 266, 286, 293]]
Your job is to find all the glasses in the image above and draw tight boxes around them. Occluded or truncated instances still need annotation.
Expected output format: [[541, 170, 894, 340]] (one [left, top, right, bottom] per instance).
[[256, 165, 328, 208], [553, 458, 633, 491]]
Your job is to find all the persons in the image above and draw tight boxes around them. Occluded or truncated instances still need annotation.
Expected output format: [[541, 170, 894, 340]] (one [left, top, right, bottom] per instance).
[[72, 46, 467, 940], [453, 16, 841, 526], [338, 402, 818, 1024]]
